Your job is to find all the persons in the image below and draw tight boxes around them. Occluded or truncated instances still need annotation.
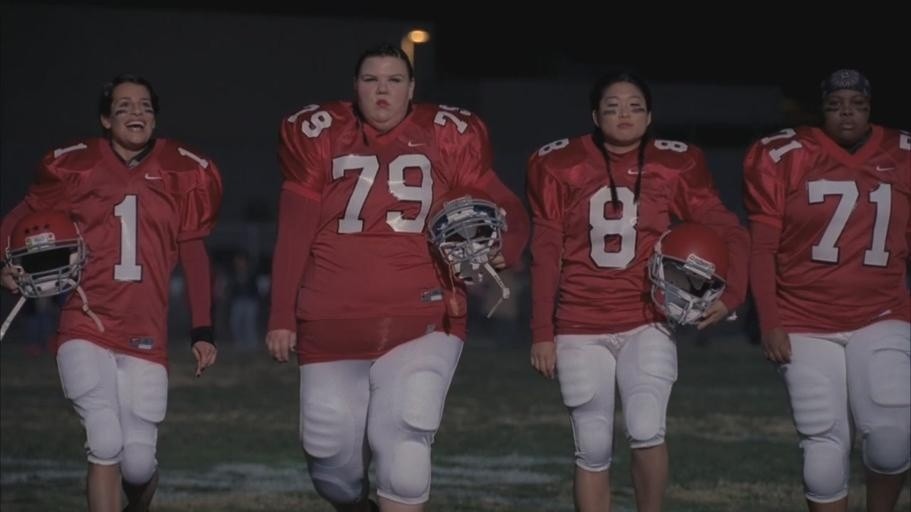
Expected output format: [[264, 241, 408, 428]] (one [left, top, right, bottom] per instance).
[[264, 33, 534, 512], [0, 68, 228, 512], [525, 64, 755, 511], [213, 249, 272, 344], [743, 46, 910, 511]]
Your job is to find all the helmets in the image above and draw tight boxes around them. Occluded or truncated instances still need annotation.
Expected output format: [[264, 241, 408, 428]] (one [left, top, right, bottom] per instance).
[[652, 220, 730, 306], [423, 185, 498, 232], [6, 210, 87, 256]]
